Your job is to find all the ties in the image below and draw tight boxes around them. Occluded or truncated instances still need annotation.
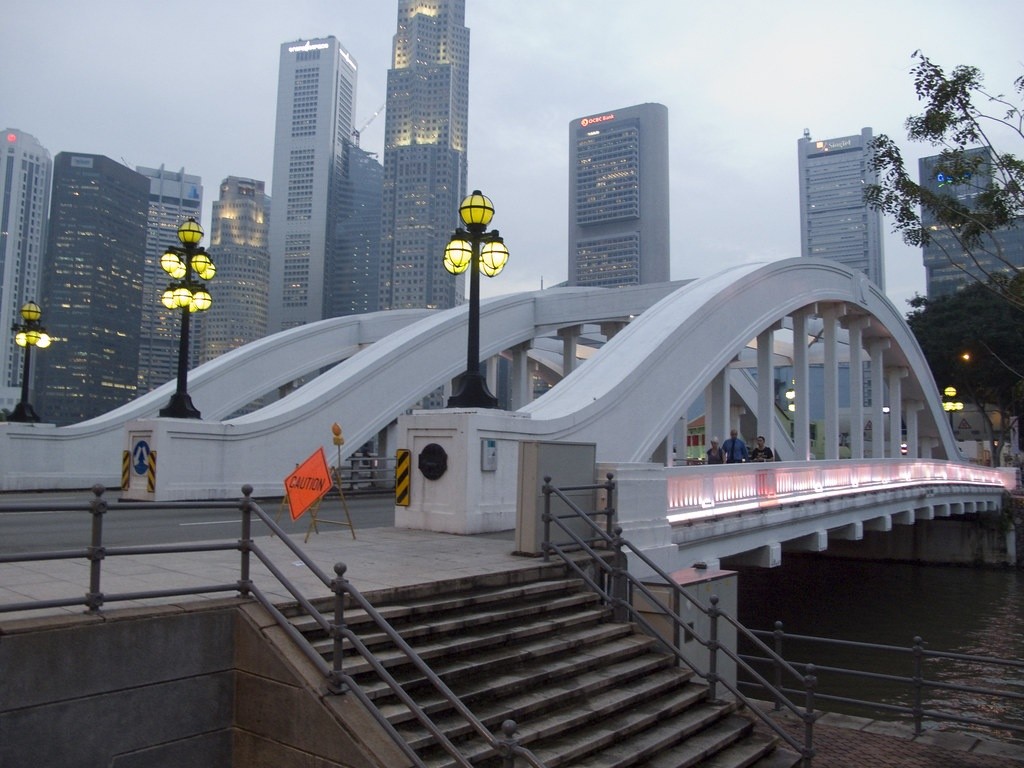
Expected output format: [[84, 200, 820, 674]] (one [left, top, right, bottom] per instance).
[[731, 440, 735, 460]]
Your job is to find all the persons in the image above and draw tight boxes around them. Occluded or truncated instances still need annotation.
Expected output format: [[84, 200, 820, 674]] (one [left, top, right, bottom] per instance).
[[722, 430, 750, 463], [750, 436, 774, 462], [706, 435, 726, 464]]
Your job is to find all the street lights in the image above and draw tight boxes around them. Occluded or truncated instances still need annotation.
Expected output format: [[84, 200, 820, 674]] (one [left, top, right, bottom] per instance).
[[943, 384, 964, 431], [160, 217, 216, 418], [442, 189, 510, 409], [7, 300, 52, 423]]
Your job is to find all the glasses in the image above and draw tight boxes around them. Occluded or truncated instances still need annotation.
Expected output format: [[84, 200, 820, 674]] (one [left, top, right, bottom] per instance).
[[731, 433, 737, 435]]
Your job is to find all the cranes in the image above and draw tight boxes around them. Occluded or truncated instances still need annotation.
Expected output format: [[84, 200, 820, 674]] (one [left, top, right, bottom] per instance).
[[352, 104, 387, 148]]
